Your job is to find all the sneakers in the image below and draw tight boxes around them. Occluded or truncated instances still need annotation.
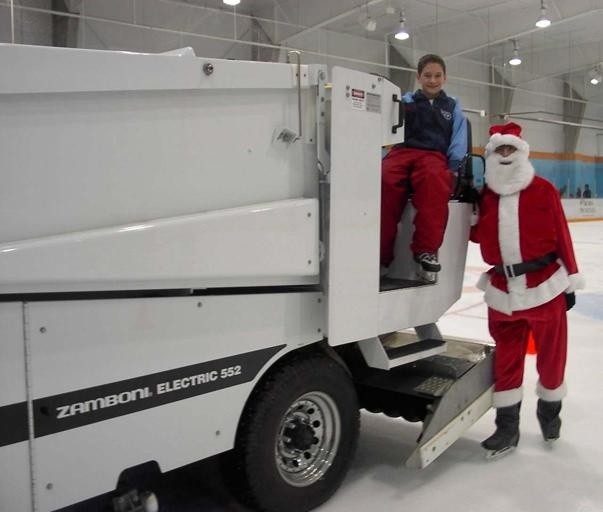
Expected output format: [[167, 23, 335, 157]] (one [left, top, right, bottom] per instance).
[[413, 251, 440, 272]]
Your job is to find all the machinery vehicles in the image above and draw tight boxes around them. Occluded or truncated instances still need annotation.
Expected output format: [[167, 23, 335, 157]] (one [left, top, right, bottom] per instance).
[[1, 43, 497, 510]]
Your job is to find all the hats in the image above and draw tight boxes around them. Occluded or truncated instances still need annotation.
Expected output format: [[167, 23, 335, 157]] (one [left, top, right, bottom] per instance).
[[484, 122, 529, 156]]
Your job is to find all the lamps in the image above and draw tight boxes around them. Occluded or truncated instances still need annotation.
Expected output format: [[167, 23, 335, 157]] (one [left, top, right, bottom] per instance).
[[393, 10, 410, 40], [508, 40, 522, 66], [223, 0, 243, 9], [535, 0, 552, 28], [590, 74, 602, 86]]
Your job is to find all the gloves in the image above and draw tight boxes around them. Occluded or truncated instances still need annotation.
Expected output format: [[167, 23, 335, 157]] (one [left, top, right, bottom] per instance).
[[567, 292, 575, 311]]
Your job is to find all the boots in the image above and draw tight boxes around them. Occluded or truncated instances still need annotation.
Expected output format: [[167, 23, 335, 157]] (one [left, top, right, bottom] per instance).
[[535, 379, 567, 440], [481, 385, 524, 450]]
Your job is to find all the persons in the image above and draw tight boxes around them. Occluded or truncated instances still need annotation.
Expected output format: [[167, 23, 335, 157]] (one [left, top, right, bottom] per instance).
[[379, 54, 469, 282], [469, 121, 586, 451], [559, 183, 592, 199]]
